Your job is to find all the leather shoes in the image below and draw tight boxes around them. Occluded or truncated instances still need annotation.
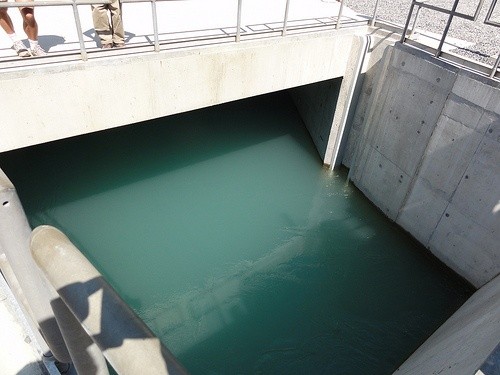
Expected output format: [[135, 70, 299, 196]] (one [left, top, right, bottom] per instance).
[[101, 43, 114, 49], [114, 43, 125, 48]]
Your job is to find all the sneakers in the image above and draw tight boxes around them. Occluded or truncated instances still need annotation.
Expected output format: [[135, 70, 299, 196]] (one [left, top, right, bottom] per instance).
[[14, 41, 30, 57], [29, 45, 48, 57]]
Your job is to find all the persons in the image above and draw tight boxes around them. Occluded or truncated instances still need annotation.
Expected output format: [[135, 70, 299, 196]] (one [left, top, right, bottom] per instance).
[[90, 0, 125, 50], [0, 0, 50, 57]]
